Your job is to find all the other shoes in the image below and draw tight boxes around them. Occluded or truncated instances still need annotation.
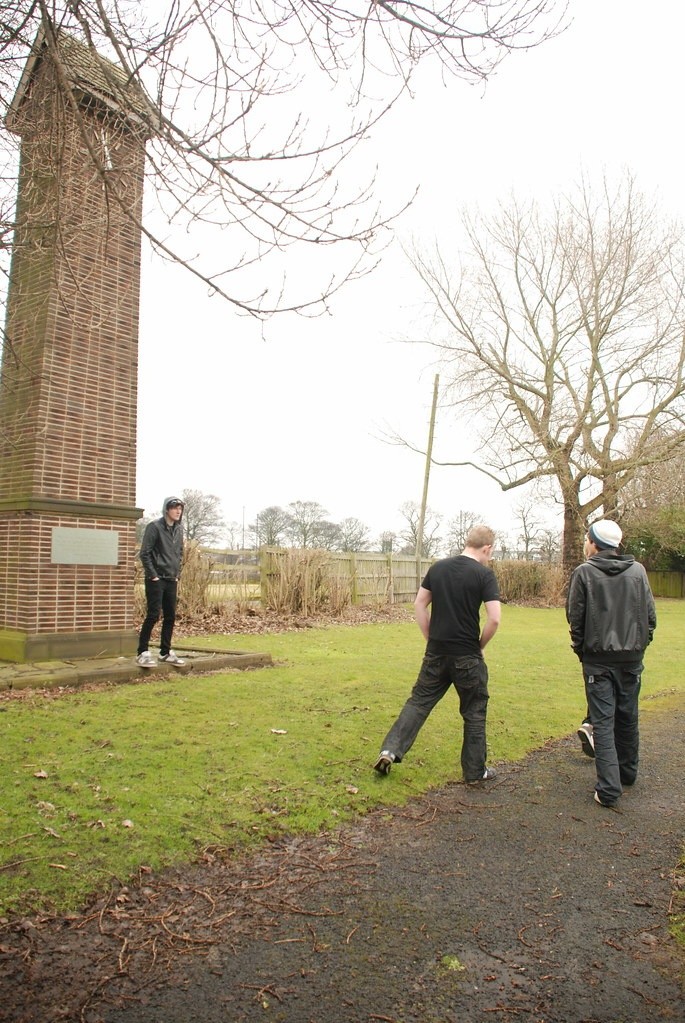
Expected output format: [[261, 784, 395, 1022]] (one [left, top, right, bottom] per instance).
[[594, 791, 617, 807]]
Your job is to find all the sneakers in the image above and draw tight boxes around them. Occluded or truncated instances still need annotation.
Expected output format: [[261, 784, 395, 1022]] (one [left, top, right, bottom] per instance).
[[373, 750, 396, 774], [159, 649, 186, 667], [136, 651, 158, 668], [577, 723, 595, 758], [466, 766, 497, 784]]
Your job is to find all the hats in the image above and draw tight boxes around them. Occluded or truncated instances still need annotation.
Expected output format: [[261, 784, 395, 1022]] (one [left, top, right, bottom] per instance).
[[588, 520, 622, 551]]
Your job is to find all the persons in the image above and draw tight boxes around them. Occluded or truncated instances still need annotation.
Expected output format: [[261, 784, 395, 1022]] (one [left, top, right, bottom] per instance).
[[133, 496, 188, 669], [564, 518, 658, 806], [578, 703, 596, 757], [366, 523, 501, 785]]
